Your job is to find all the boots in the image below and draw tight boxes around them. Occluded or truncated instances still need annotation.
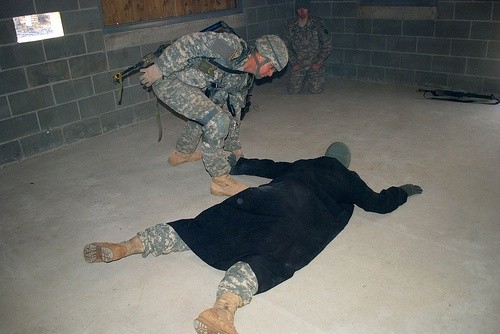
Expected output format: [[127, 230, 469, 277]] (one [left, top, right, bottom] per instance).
[[84, 237, 144, 263], [193, 293, 242, 334]]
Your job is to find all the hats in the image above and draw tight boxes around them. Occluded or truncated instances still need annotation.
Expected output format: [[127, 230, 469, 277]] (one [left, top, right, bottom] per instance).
[[326, 141, 352, 168]]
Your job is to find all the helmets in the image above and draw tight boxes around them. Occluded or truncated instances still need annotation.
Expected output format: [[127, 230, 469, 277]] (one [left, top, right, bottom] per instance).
[[255, 34, 288, 72], [295, 0, 311, 11]]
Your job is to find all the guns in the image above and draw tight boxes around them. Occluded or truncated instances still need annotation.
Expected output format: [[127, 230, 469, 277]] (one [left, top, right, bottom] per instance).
[[416, 86, 500, 106], [112, 19, 234, 83]]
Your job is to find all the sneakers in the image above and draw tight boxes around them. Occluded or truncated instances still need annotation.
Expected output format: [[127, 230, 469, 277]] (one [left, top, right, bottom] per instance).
[[210, 177, 247, 197], [168, 150, 202, 166]]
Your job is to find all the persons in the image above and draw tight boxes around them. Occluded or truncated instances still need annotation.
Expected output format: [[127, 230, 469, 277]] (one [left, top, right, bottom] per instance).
[[139, 31, 288, 197], [83, 142, 424, 334], [279, 0, 332, 94]]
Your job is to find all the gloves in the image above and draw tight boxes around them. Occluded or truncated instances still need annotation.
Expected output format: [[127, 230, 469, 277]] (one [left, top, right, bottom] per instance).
[[139, 64, 162, 87], [227, 153, 237, 168], [399, 184, 423, 196], [232, 149, 244, 162]]
